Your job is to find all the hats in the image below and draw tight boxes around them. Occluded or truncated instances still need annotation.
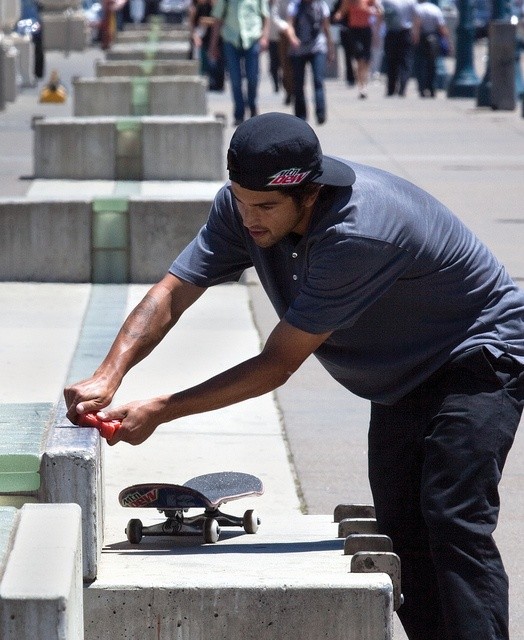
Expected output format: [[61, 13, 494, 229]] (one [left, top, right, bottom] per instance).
[[228, 113, 357, 188]]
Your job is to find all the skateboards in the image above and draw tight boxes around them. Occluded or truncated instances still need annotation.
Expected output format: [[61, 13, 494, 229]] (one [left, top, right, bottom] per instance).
[[118, 471, 264, 544]]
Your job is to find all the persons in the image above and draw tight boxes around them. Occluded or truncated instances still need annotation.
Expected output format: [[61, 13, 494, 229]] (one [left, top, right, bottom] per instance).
[[274, 2, 336, 126], [332, 0, 384, 100], [185, 1, 208, 60], [208, 0, 271, 126], [61, 113, 524, 632], [416, 3, 450, 101], [379, 0, 424, 99], [203, 23, 227, 91]]
